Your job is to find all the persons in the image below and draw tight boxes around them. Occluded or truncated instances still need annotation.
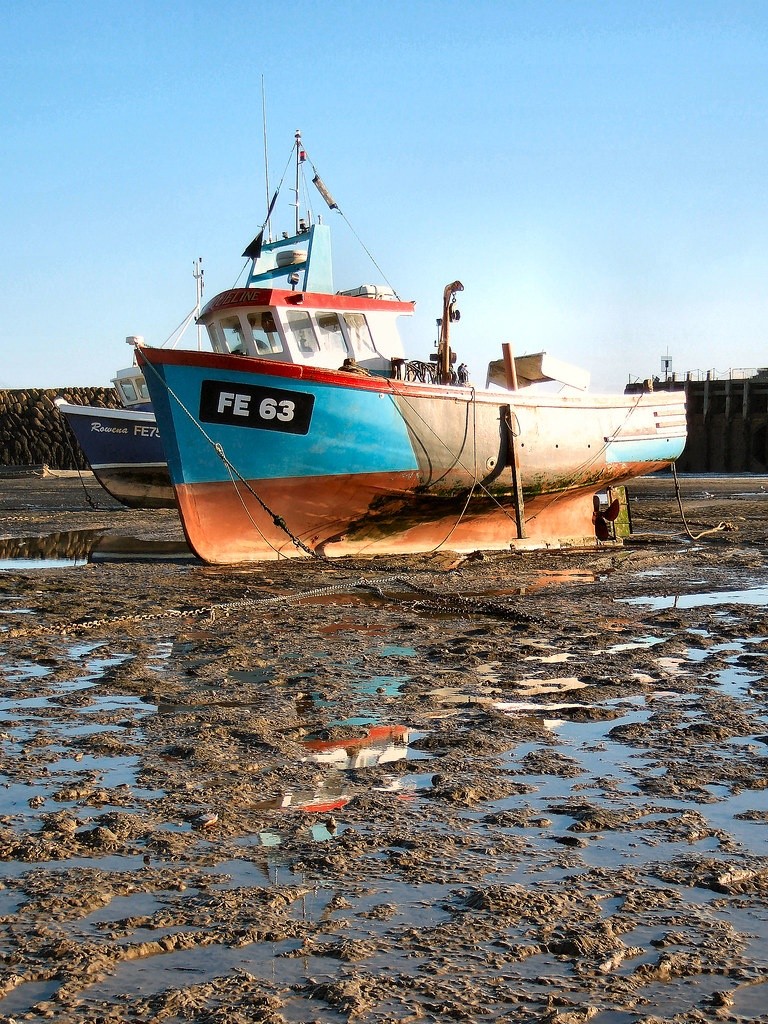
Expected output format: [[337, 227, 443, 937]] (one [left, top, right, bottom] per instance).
[[457, 363, 469, 384]]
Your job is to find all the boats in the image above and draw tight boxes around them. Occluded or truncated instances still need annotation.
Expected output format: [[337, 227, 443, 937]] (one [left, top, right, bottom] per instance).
[[55, 255, 204, 509], [129, 72, 687, 567]]
